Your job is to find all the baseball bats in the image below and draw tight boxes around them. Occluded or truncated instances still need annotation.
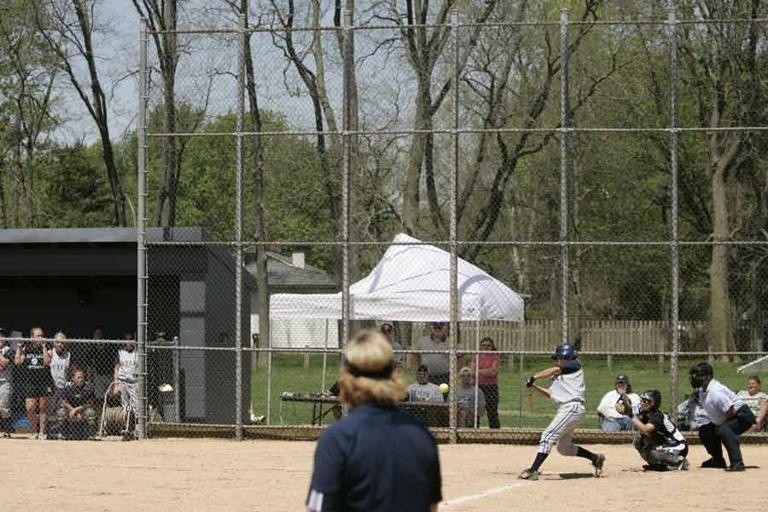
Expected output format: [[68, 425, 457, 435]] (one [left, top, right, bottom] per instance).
[[526, 387, 535, 411]]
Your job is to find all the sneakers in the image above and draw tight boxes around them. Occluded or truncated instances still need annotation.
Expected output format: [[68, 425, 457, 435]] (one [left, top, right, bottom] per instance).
[[517, 468, 537, 480], [725, 462, 744, 472], [701, 458, 727, 468], [592, 453, 605, 478]]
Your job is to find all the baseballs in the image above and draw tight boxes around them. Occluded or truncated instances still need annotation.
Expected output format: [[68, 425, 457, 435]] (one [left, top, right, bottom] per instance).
[[439, 383, 449, 393]]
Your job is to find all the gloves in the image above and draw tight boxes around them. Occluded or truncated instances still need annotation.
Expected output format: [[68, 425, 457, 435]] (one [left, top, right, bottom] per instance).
[[525, 376, 534, 388]]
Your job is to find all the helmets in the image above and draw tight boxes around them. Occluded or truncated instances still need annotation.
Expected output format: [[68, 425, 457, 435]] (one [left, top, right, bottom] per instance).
[[551, 344, 577, 366], [637, 389, 661, 414], [688, 362, 713, 388]]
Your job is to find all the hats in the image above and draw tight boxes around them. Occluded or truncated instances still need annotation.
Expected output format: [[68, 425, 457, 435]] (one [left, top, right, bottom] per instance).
[[616, 374, 628, 384], [460, 368, 471, 376]]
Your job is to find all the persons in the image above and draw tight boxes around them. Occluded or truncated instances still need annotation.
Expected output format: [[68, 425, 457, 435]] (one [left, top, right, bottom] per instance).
[[515, 343, 605, 481], [410, 322, 467, 426], [736, 374, 768, 434], [676, 386, 712, 432], [445, 366, 487, 427], [0, 326, 173, 440], [380, 323, 406, 376], [304, 328, 442, 512], [405, 364, 443, 427], [596, 373, 644, 434], [689, 362, 756, 472], [614, 389, 690, 471], [471, 337, 501, 429], [326, 382, 344, 421]]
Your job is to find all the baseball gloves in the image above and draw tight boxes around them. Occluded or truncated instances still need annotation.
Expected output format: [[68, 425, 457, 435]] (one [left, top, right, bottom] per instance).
[[615, 394, 634, 417]]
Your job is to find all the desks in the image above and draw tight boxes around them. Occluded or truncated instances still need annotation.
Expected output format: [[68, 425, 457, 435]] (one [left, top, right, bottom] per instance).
[[281, 394, 461, 429]]
